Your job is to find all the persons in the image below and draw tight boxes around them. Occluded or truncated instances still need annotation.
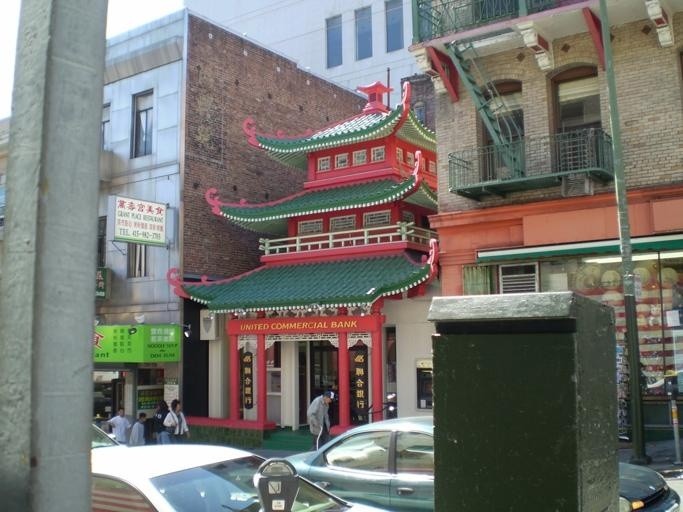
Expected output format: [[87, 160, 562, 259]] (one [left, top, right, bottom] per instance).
[[162, 399, 190, 439], [128, 412, 147, 445], [153, 400, 170, 443], [106, 407, 131, 444], [305, 391, 334, 452]]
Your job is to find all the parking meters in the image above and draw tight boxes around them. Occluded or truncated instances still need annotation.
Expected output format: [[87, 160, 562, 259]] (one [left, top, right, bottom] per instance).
[[663, 375, 682, 465], [252, 456, 300, 512]]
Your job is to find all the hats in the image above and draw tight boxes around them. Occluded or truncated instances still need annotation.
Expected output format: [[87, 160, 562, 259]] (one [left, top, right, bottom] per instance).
[[323, 390, 335, 400]]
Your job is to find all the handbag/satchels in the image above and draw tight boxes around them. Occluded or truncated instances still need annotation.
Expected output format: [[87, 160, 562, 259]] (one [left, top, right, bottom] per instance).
[[165, 411, 176, 435]]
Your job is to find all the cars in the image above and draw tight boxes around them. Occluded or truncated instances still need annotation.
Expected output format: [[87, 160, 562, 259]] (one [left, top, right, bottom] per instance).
[[90, 441, 392, 512], [646, 371, 683, 395], [91, 423, 129, 450], [286, 414, 682, 512]]
